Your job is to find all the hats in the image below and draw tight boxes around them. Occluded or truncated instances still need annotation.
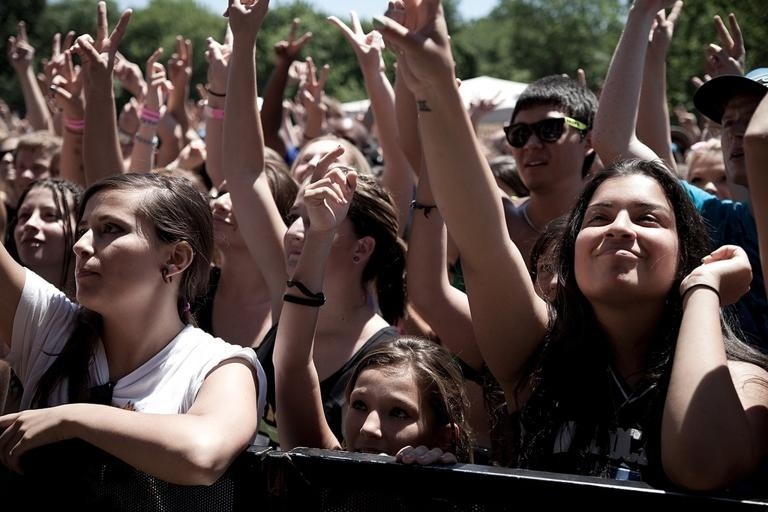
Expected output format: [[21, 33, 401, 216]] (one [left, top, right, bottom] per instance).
[[693, 67, 768, 125]]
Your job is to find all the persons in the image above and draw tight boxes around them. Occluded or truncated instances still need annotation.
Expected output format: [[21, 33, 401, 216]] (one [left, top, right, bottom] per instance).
[[0, 1, 768, 512]]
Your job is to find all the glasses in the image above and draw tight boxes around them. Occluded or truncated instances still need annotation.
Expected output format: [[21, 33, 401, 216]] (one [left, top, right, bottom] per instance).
[[503, 117, 587, 149]]
